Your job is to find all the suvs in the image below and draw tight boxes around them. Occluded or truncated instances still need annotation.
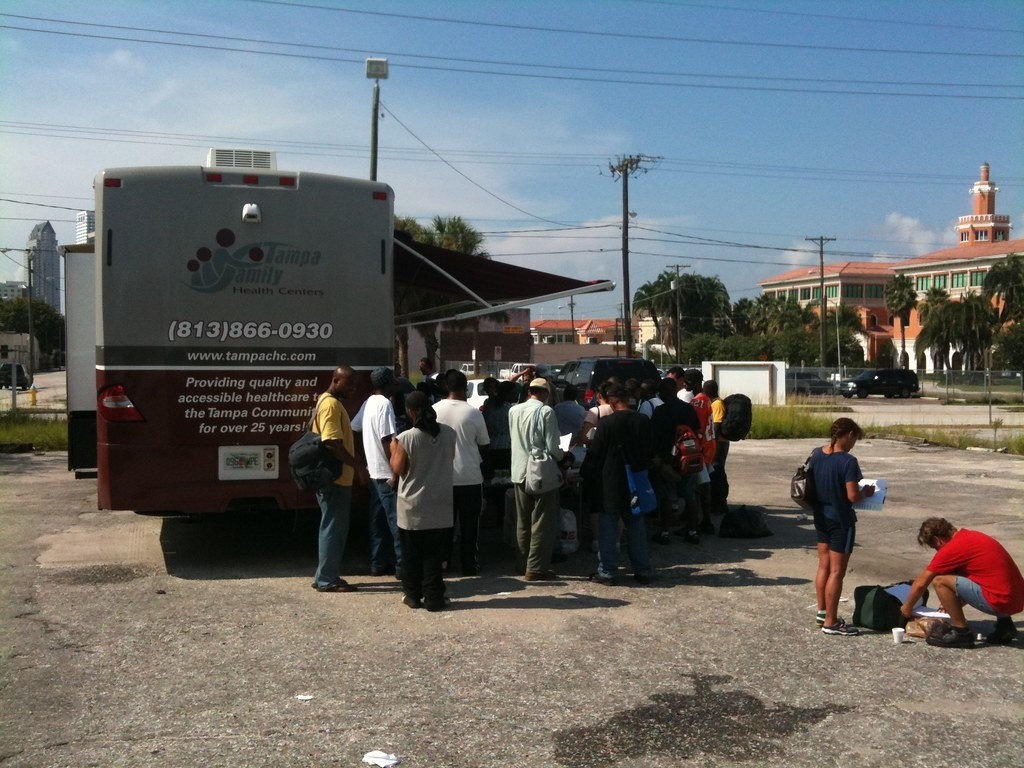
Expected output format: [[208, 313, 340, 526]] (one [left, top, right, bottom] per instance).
[[554, 354, 662, 410], [836, 368, 919, 400], [0, 362, 33, 391]]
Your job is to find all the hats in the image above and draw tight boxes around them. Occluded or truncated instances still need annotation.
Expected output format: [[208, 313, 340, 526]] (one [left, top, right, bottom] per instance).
[[530, 378, 550, 394]]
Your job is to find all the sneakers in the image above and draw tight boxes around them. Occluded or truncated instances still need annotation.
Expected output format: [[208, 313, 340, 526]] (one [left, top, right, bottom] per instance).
[[987, 617, 1018, 644], [926, 629, 975, 649]]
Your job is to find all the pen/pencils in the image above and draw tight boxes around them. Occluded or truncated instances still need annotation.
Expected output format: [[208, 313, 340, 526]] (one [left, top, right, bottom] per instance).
[[870, 482, 874, 486], [937, 607, 941, 612]]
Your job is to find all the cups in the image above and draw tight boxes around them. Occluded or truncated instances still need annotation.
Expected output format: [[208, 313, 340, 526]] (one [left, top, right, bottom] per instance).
[[892, 628, 905, 643]]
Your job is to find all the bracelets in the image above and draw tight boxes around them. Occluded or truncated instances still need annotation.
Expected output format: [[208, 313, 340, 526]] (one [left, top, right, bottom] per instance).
[[352, 459, 356, 467]]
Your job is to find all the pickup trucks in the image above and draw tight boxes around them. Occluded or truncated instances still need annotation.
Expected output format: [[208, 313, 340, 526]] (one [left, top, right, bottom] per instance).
[[501, 363, 537, 383]]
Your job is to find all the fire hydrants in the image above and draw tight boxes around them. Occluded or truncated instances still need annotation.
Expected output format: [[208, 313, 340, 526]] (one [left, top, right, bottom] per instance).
[[27, 385, 38, 405]]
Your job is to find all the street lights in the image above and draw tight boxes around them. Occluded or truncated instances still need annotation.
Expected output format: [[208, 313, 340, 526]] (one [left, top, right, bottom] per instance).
[[808, 270, 825, 368], [367, 59, 388, 182], [17, 283, 34, 369], [622, 210, 637, 358]]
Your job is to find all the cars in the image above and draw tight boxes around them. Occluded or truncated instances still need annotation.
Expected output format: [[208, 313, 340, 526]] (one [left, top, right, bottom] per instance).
[[459, 363, 477, 376], [785, 372, 835, 396], [465, 377, 489, 410]]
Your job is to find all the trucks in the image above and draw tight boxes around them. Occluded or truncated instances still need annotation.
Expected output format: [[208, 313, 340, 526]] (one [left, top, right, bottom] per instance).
[[57, 145, 394, 517]]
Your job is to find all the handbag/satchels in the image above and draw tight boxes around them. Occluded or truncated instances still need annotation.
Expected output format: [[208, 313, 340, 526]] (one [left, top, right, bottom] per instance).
[[906, 617, 951, 638], [289, 430, 343, 491], [852, 580, 929, 629], [791, 448, 816, 511], [626, 465, 659, 516], [525, 450, 563, 495]]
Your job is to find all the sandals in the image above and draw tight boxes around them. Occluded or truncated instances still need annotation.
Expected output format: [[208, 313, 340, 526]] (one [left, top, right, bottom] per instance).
[[821, 617, 859, 635], [816, 611, 845, 626]]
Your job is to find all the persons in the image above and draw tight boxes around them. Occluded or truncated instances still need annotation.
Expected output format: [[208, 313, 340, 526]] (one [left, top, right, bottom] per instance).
[[808, 417, 875, 636], [312, 366, 370, 592], [351, 357, 728, 580], [901, 516, 1024, 649], [390, 391, 456, 612]]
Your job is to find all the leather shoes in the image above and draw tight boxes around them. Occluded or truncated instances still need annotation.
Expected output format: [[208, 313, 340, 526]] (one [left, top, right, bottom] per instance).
[[588, 573, 617, 586], [525, 569, 556, 580], [634, 571, 649, 585]]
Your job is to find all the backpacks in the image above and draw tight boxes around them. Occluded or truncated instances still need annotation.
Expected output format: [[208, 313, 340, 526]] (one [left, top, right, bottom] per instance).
[[721, 393, 752, 441], [671, 424, 705, 475]]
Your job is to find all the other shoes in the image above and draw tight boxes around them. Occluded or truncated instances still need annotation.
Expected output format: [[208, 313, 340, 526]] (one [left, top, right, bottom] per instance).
[[655, 534, 670, 545], [682, 535, 700, 545], [403, 595, 420, 609], [424, 597, 450, 611], [312, 578, 358, 592]]
